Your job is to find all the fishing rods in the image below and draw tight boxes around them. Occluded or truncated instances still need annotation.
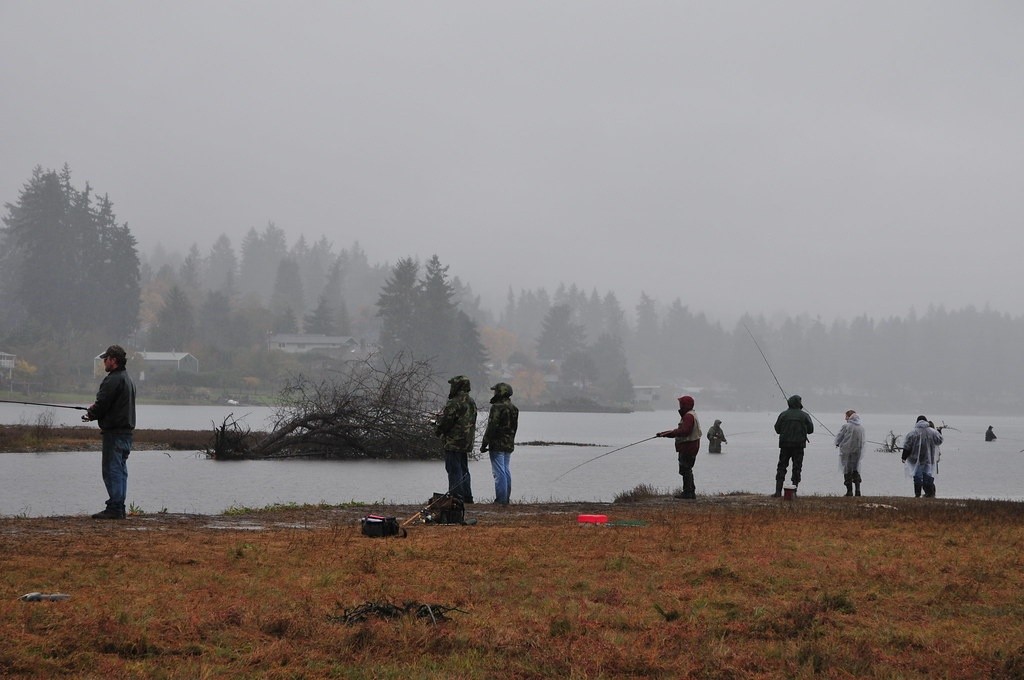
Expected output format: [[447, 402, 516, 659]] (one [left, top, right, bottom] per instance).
[[743, 323, 812, 445], [0, 400, 89, 411], [712, 431, 755, 437], [556, 431, 672, 480], [403, 472, 471, 525], [802, 406, 835, 437], [355, 397, 441, 426]]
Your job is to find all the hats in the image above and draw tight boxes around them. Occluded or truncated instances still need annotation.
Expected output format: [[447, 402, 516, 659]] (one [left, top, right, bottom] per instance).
[[678, 396, 694, 408], [489, 382, 513, 404], [100, 345, 126, 358], [448, 376, 471, 398], [787, 395, 803, 409]]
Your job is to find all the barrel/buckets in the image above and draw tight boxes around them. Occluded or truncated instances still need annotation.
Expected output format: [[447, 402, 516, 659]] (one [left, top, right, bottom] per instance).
[[784, 485, 797, 500]]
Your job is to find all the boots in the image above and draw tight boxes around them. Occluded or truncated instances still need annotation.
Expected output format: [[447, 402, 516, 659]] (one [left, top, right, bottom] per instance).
[[771, 480, 783, 497], [844, 483, 860, 496]]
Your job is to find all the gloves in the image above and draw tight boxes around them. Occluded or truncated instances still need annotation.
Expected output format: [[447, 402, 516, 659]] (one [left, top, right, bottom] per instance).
[[435, 430, 441, 437], [480, 444, 487, 453]]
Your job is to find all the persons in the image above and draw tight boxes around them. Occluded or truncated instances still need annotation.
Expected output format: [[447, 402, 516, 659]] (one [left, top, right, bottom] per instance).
[[660, 396, 702, 499], [985, 426, 996, 441], [835, 410, 865, 497], [81, 344, 136, 520], [901, 416, 943, 498], [707, 420, 727, 454], [480, 382, 519, 505], [435, 376, 478, 504], [771, 395, 814, 498]]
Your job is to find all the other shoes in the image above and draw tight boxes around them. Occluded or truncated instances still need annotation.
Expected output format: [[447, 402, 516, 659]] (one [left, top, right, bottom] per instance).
[[91, 503, 126, 521], [674, 490, 696, 499]]
